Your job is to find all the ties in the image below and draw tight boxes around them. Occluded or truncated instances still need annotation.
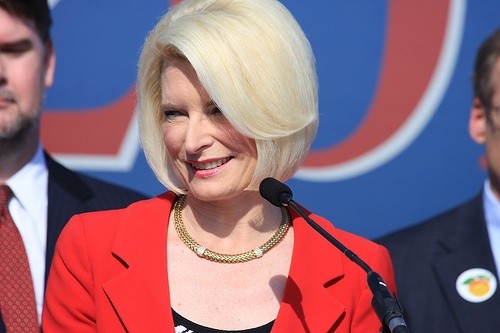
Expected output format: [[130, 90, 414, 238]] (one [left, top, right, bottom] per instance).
[[0, 183, 41, 333]]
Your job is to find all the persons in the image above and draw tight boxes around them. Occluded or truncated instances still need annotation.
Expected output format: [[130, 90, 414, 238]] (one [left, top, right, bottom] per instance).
[[38, 1, 396, 332], [2, 1, 150, 332], [372, 28, 500, 333]]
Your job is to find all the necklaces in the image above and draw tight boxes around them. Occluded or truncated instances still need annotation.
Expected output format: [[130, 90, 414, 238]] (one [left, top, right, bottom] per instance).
[[173, 192, 292, 266]]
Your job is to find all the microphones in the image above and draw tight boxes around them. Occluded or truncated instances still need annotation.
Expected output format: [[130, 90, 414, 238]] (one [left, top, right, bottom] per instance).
[[259, 176, 413, 333]]
